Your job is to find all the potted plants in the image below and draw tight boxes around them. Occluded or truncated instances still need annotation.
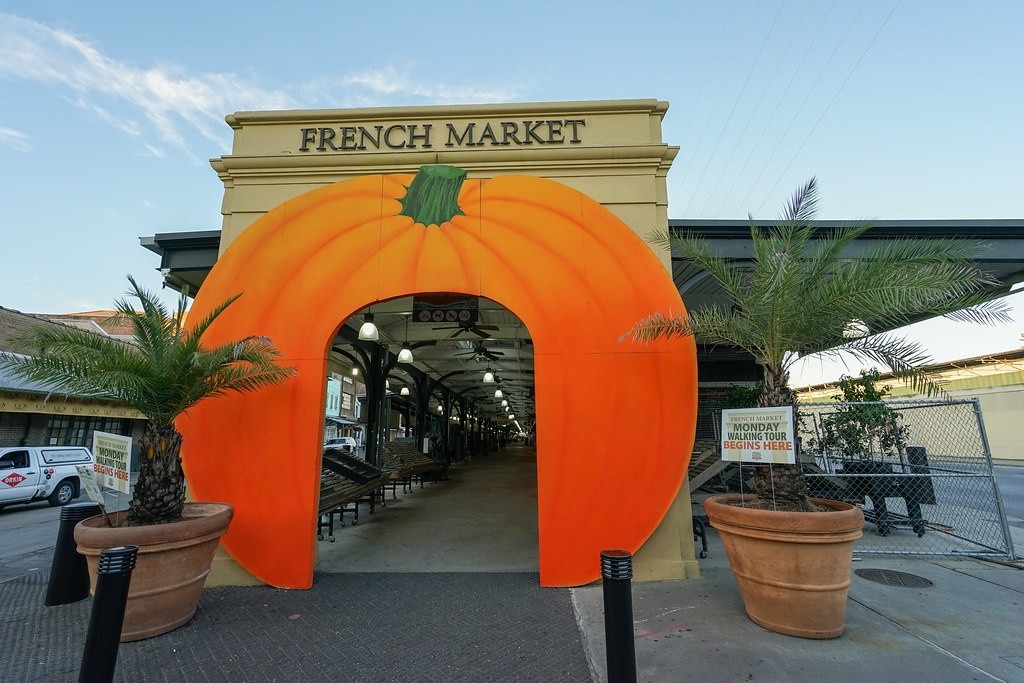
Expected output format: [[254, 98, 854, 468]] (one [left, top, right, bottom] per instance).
[[616, 174, 1012, 641], [0, 277, 296, 640], [809, 368, 912, 480]]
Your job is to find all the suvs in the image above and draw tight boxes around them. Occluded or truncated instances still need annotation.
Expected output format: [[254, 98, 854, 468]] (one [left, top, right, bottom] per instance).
[[324, 438, 357, 454]]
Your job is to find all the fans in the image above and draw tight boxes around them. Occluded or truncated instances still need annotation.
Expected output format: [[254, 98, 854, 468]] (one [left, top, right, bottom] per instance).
[[479, 370, 514, 383], [431, 322, 501, 338], [453, 341, 505, 363]]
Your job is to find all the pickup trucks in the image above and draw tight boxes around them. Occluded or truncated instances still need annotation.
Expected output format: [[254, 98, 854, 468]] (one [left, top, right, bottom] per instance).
[[0, 446, 97, 507]]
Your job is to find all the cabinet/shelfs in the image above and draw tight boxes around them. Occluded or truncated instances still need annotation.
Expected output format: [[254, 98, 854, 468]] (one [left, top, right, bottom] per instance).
[[317, 441, 431, 542]]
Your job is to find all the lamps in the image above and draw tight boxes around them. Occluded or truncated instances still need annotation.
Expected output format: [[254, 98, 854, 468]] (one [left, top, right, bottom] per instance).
[[401, 376, 409, 396], [494, 386, 503, 397], [358, 307, 380, 341], [385, 378, 389, 388], [501, 398, 508, 407], [397, 316, 414, 363], [482, 360, 494, 383], [352, 352, 358, 376], [437, 403, 525, 438]]
